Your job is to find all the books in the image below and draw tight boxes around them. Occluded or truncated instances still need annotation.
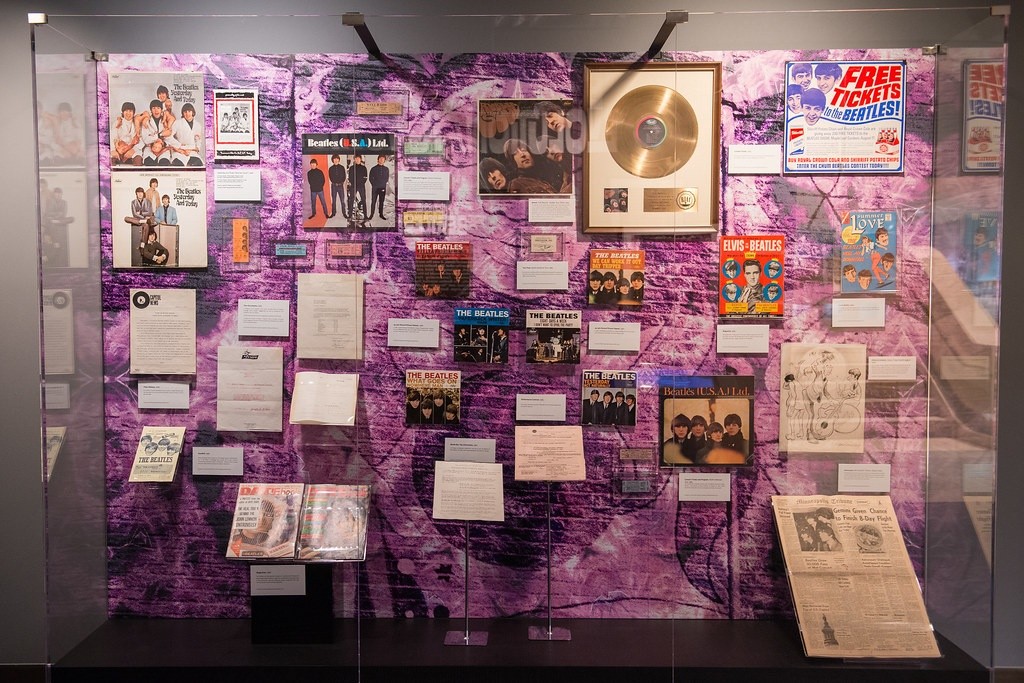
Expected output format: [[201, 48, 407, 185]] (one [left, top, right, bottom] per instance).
[[293, 482, 372, 560], [226, 481, 305, 561]]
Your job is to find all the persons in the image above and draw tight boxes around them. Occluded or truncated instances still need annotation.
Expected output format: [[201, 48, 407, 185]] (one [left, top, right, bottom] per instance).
[[455, 327, 508, 364], [124, 178, 177, 266], [789, 61, 842, 125], [231, 500, 274, 555], [582, 389, 637, 425], [480, 100, 574, 194], [307, 152, 390, 220], [722, 258, 780, 318], [140, 436, 178, 456], [664, 413, 750, 465], [588, 269, 644, 306], [604, 189, 629, 212], [111, 84, 206, 167], [794, 508, 843, 550], [423, 262, 467, 298], [406, 388, 460, 423], [531, 337, 580, 363]]
